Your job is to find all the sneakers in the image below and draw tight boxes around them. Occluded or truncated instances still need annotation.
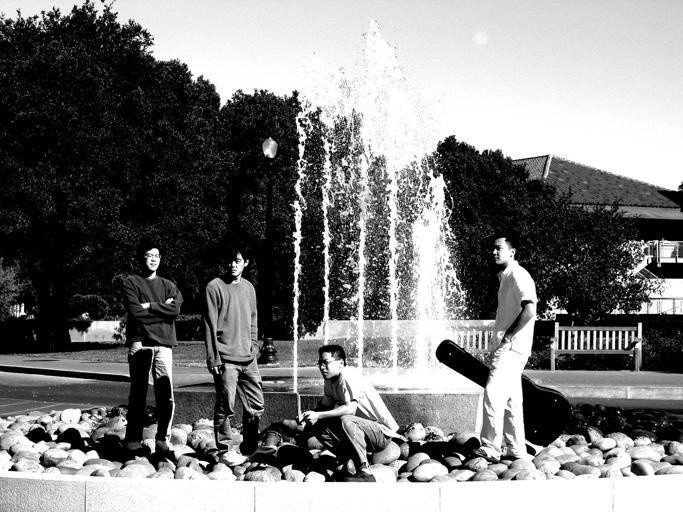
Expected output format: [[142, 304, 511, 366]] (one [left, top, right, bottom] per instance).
[[242, 445, 277, 459], [128, 439, 143, 449], [219, 449, 249, 466], [473, 447, 500, 464], [501, 447, 527, 459], [155, 440, 169, 452]]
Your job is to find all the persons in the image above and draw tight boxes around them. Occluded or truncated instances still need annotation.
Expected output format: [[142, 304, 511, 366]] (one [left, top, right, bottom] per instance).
[[120, 238, 185, 457], [201, 242, 267, 468], [471, 234, 538, 465], [295, 344, 401, 482]]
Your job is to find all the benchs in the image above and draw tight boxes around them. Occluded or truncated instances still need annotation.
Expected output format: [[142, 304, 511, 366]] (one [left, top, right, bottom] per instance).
[[444, 324, 494, 368], [550, 321, 643, 372]]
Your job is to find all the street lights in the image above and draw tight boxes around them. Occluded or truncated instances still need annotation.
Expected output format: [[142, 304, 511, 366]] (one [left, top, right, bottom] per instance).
[[254, 136, 285, 370]]
